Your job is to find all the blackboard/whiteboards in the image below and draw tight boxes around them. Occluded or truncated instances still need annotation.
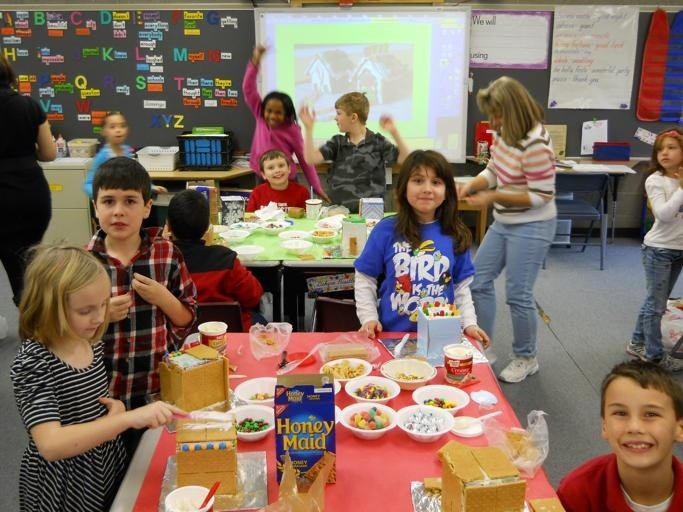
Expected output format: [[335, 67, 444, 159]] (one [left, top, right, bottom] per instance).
[[0, 0, 683, 174]]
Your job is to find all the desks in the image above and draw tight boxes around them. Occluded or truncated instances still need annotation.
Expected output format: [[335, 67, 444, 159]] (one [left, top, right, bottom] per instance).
[[159, 210, 398, 330], [105, 330, 572, 511], [466, 156, 640, 247], [130, 158, 257, 206], [446, 190, 488, 245]]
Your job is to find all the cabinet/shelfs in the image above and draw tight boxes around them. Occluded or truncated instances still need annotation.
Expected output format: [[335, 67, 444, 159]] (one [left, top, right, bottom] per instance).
[[34, 153, 99, 256], [552, 191, 574, 247]]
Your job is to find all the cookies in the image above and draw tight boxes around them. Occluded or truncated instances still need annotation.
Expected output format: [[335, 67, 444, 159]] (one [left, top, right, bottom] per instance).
[[423, 440, 566, 512], [304, 451, 336, 484]]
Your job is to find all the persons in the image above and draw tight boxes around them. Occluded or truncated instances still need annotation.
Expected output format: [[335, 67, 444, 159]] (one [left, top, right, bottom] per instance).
[[80, 113, 166, 203], [240, 38, 332, 204], [74, 155, 201, 454], [353, 150, 491, 350], [297, 90, 408, 212], [623, 125, 683, 373], [7, 237, 190, 511], [168, 190, 266, 333], [452, 74, 558, 385], [0, 54, 56, 310], [555, 361, 683, 512], [245, 150, 310, 211]]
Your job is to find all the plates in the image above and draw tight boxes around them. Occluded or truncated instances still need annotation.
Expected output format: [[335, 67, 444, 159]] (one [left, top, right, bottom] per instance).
[[254, 208, 290, 219], [225, 376, 279, 440], [208, 219, 290, 245], [285, 216, 346, 251], [321, 357, 474, 444]]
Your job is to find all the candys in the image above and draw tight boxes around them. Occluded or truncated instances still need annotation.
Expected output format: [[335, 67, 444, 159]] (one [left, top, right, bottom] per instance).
[[354, 382, 390, 399], [423, 397, 456, 409], [423, 300, 460, 318], [250, 392, 273, 400], [349, 406, 391, 430], [236, 417, 269, 432]]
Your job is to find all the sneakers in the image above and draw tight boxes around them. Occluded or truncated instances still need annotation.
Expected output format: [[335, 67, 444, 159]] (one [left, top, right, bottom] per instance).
[[496, 352, 541, 386], [484, 348, 498, 365], [624, 336, 682, 374]]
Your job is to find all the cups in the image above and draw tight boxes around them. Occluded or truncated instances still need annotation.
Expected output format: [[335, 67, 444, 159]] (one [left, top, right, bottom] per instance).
[[441, 342, 475, 385], [196, 320, 228, 358], [477, 140, 489, 157], [306, 198, 321, 218], [342, 216, 367, 258], [165, 485, 215, 511]]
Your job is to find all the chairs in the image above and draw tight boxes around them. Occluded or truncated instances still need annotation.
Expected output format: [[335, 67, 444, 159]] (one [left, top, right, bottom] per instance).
[[307, 292, 360, 333], [194, 300, 244, 333], [540, 169, 613, 271]]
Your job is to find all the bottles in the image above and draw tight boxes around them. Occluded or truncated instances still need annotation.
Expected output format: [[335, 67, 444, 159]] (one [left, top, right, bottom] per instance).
[[52, 134, 67, 151]]
